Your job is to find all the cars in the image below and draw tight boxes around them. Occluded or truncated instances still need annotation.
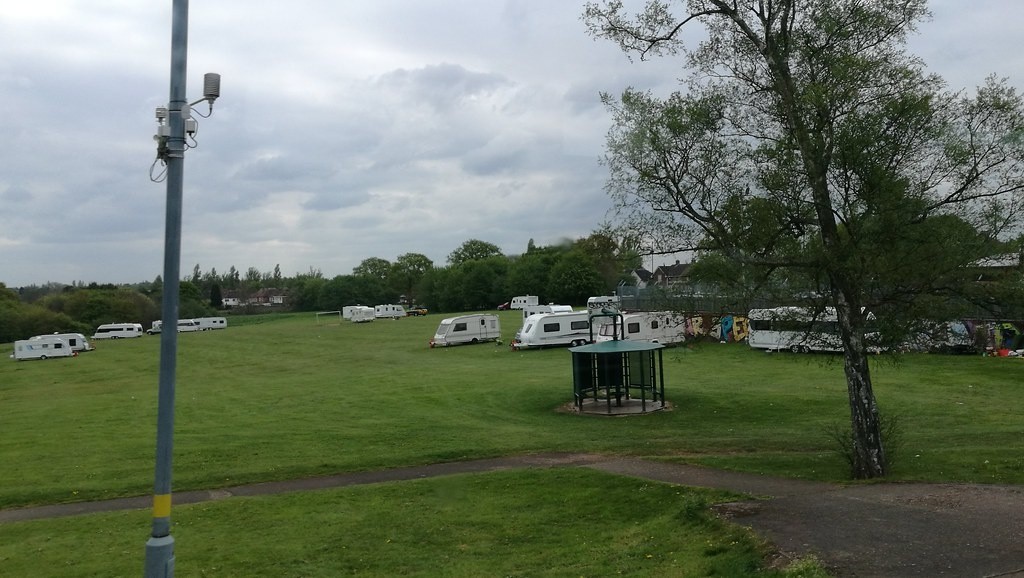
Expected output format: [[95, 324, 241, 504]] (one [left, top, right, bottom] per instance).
[[405, 304, 428, 317], [146, 328, 154, 335], [498, 302, 511, 311]]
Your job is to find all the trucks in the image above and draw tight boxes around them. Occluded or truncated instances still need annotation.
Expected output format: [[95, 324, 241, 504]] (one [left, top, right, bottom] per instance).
[[596, 311, 687, 350], [586, 295, 623, 317], [511, 308, 590, 350], [190, 316, 228, 332], [748, 305, 890, 356], [433, 314, 502, 348], [342, 303, 376, 323], [898, 316, 1021, 357], [29, 333, 89, 353], [374, 304, 407, 320], [14, 337, 73, 361], [510, 294, 539, 311], [522, 302, 574, 328], [91, 322, 144, 340], [152, 317, 199, 333], [221, 294, 289, 309]]
